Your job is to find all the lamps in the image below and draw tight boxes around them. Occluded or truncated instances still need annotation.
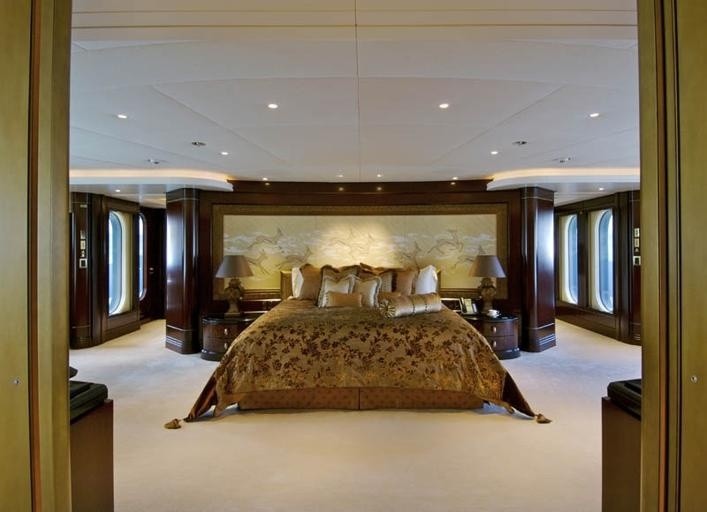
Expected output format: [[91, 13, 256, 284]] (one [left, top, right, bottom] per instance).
[[468, 254, 509, 316], [215, 255, 253, 317]]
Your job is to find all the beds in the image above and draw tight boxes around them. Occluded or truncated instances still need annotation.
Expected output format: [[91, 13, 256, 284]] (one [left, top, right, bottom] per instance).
[[183, 269, 536, 422]]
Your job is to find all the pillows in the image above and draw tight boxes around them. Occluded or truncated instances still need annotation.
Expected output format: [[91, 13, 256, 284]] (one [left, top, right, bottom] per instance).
[[289, 260, 442, 316]]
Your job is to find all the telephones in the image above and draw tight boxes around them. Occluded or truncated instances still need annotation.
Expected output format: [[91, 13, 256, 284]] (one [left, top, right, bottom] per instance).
[[460, 297, 477, 315]]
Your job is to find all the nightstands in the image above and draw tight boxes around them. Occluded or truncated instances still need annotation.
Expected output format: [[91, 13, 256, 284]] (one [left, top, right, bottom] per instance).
[[200, 316, 255, 362], [468, 313, 520, 359]]
